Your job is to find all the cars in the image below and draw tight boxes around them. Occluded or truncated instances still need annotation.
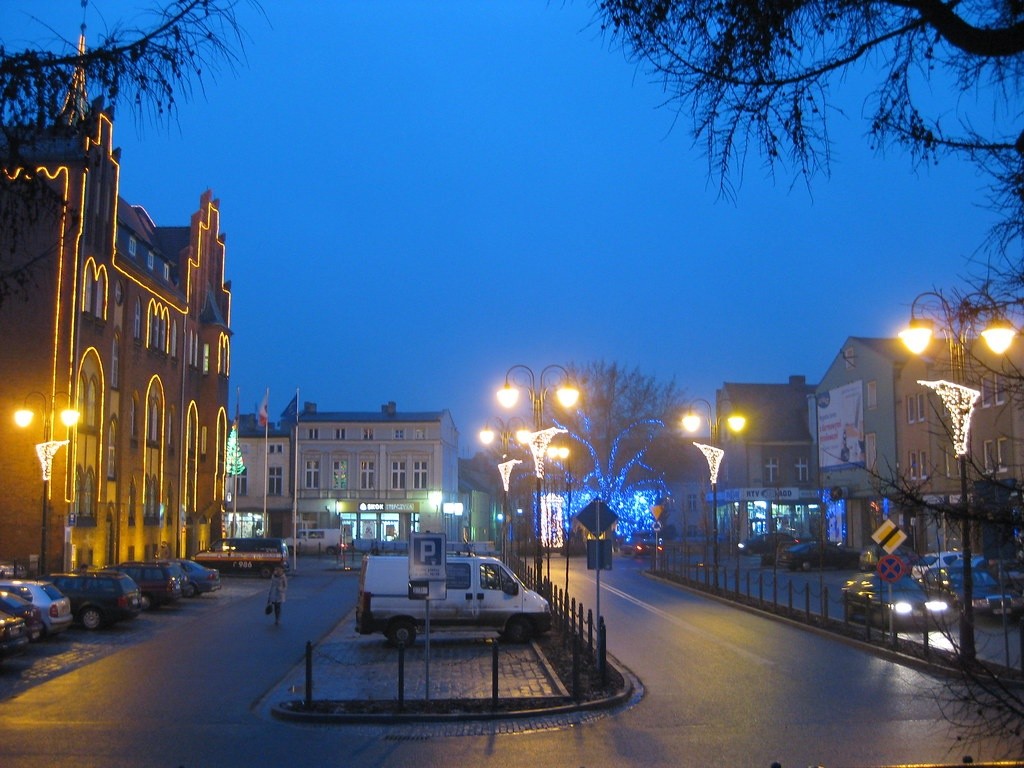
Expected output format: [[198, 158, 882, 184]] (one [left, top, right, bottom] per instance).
[[918, 565, 1024, 620], [738, 533, 801, 556], [0, 609, 30, 660], [840, 566, 947, 634], [621, 538, 661, 558], [860, 542, 1024, 589], [0, 590, 45, 641], [159, 558, 221, 598], [785, 539, 862, 572]]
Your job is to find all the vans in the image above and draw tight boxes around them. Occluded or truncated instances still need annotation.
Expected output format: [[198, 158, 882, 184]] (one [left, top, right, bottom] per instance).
[[284, 528, 342, 556], [353, 552, 555, 648], [191, 537, 290, 579]]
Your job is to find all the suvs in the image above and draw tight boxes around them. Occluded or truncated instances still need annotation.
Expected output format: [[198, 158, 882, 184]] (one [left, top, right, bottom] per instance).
[[30, 570, 142, 632], [0, 575, 74, 638], [101, 560, 183, 610]]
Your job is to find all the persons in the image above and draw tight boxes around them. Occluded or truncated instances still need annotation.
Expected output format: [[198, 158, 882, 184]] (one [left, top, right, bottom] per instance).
[[463, 526, 469, 545], [266, 567, 288, 626]]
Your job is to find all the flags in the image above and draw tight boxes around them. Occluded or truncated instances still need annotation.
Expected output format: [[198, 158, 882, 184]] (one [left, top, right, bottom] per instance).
[[273, 395, 298, 435], [258, 395, 267, 427], [231, 402, 239, 430]]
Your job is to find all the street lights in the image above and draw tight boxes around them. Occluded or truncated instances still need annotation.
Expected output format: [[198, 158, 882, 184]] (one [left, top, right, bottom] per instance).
[[898, 291, 1024, 671], [14, 390, 81, 583], [478, 415, 530, 562], [498, 363, 587, 589], [683, 398, 747, 596]]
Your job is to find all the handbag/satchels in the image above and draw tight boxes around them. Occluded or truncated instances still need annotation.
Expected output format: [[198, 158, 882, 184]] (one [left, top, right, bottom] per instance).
[[265, 602, 274, 614]]
